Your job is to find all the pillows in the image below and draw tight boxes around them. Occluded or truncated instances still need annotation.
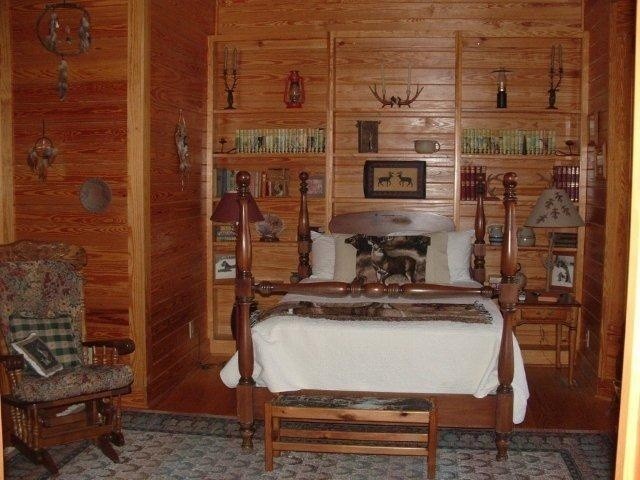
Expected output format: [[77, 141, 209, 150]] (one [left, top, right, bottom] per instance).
[[5, 309, 82, 378], [305, 224, 475, 286]]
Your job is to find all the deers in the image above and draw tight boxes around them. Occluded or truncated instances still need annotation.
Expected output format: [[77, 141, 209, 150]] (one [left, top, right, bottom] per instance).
[[377, 171, 396, 187], [361, 234, 417, 286], [395, 170, 413, 187]]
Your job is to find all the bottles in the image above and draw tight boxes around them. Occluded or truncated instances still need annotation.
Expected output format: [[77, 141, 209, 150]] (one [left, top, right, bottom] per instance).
[[487, 225, 503, 246], [515, 225, 536, 247]]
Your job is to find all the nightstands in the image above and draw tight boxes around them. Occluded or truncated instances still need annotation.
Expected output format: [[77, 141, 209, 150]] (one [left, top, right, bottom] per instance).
[[513, 292, 581, 382]]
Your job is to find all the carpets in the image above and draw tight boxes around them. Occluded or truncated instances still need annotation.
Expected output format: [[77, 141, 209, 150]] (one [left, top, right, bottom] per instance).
[[5, 404, 616, 480]]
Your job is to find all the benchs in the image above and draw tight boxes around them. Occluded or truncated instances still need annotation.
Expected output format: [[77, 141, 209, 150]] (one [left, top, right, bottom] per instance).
[[263, 390, 437, 480]]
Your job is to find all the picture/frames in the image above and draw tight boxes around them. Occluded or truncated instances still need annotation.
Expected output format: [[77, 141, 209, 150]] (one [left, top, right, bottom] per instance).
[[362, 159, 427, 199], [552, 251, 578, 293]]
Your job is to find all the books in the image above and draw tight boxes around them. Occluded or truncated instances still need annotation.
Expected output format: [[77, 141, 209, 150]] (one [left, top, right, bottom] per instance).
[[212, 169, 290, 196], [461, 128, 558, 156], [461, 165, 486, 200], [553, 165, 579, 203], [236, 128, 326, 153]]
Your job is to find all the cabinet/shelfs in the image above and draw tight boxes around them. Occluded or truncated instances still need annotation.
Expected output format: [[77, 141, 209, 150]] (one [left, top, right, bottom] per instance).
[[205, 26, 590, 363]]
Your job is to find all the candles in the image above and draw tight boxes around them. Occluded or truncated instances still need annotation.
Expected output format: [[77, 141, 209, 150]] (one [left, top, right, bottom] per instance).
[[224, 45, 237, 72], [550, 43, 563, 72]]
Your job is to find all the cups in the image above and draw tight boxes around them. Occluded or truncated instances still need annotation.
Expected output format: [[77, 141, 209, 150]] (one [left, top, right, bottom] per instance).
[[412, 140, 441, 153]]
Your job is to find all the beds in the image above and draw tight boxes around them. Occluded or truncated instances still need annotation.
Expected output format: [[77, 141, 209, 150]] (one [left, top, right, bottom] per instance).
[[237, 169, 520, 462]]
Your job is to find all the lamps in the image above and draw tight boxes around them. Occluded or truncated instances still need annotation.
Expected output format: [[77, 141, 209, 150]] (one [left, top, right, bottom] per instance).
[[283, 69, 306, 108], [490, 67, 513, 108], [525, 187, 585, 301], [209, 192, 265, 340]]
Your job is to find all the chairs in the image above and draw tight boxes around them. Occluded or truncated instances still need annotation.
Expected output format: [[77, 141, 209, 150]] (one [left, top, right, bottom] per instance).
[[0, 239, 137, 475]]
